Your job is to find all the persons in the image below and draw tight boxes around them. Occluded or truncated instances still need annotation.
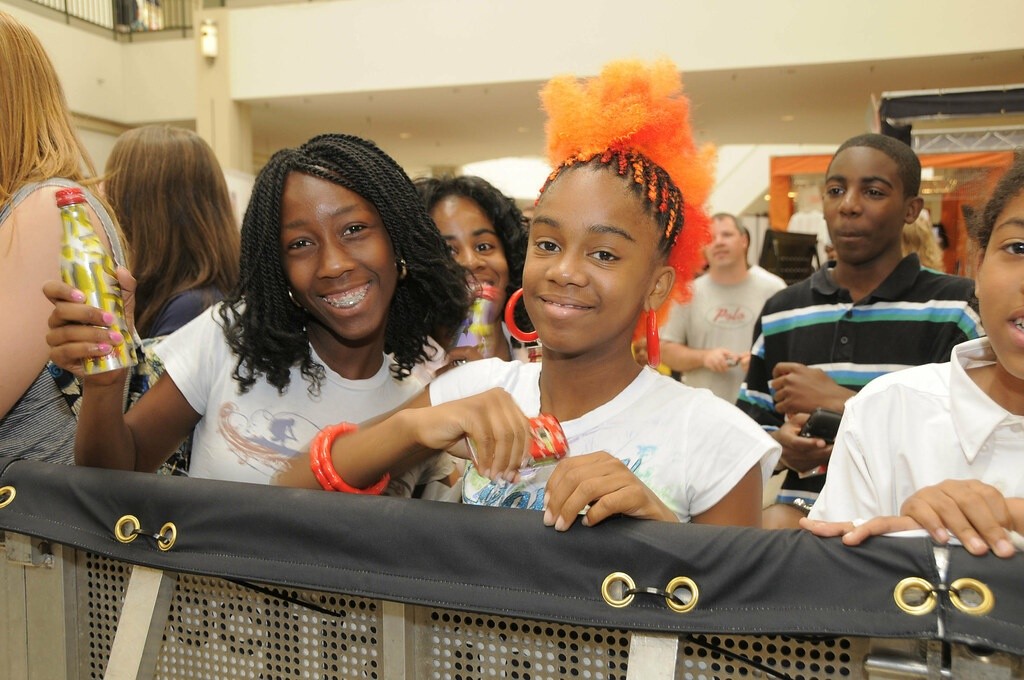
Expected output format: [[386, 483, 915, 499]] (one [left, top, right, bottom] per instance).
[[800, 164, 1024, 557], [269, 57, 783, 531], [104, 125, 240, 476], [42, 133, 510, 506], [656, 213, 787, 406], [736, 135, 986, 530], [1, 10, 127, 469], [414, 177, 543, 348]]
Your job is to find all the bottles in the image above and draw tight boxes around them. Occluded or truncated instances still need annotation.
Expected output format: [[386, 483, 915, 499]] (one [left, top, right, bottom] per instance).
[[448, 287, 499, 373], [51, 188, 139, 374]]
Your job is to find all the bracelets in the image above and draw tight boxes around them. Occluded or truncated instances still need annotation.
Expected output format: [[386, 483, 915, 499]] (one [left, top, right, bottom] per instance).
[[310, 422, 390, 494]]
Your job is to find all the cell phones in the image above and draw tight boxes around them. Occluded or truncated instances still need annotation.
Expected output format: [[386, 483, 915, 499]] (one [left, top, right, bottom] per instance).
[[799, 410, 843, 446]]
